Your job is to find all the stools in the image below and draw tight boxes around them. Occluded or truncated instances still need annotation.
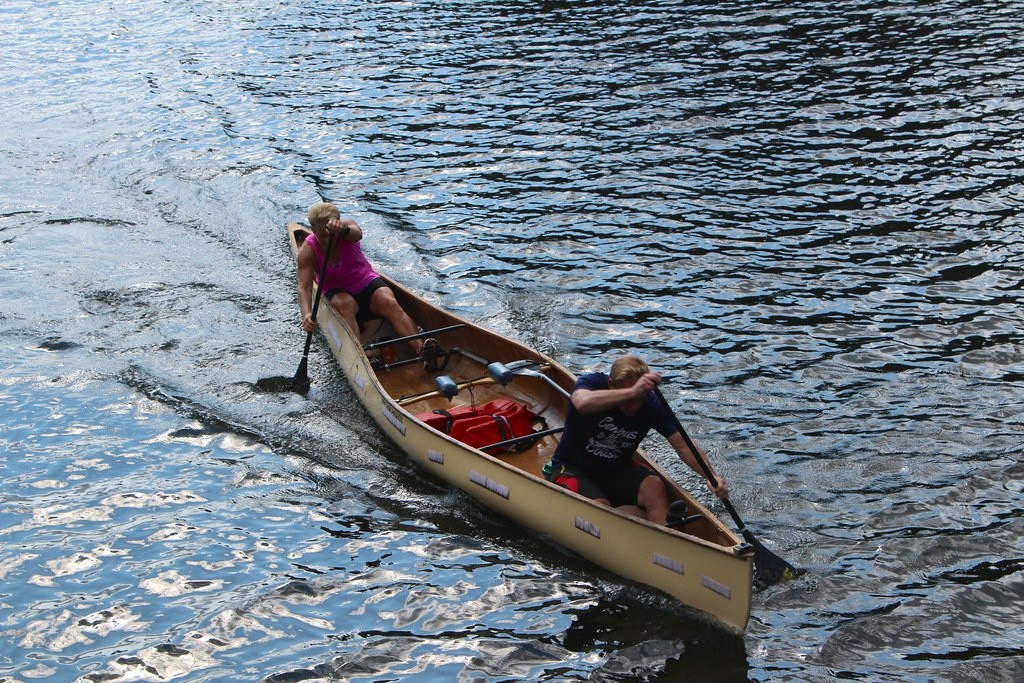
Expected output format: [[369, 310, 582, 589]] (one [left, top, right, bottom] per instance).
[[356, 284, 402, 345]]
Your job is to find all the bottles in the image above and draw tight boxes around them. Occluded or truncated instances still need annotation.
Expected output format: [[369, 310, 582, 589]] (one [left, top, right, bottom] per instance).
[[541, 460, 561, 482], [553, 476, 578, 493]]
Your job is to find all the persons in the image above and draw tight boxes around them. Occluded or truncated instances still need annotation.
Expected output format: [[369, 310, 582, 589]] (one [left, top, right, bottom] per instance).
[[543, 356, 730, 526], [297, 203, 442, 374]]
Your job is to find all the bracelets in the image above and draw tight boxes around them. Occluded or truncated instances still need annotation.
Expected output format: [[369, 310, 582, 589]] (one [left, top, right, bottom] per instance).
[[342, 225, 351, 236]]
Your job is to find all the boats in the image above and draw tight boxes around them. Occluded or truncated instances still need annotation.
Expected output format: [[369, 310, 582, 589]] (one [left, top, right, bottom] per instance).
[[287, 220, 755, 640]]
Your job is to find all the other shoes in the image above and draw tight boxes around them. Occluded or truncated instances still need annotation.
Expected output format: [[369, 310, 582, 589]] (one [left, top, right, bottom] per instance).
[[418, 338, 440, 371]]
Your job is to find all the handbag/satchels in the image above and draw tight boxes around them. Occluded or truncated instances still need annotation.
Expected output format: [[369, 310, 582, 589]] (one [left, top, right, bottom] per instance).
[[449, 399, 548, 456]]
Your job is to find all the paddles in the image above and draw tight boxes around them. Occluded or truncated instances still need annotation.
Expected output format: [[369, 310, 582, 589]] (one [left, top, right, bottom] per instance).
[[293, 223, 334, 379], [651, 380, 798, 579], [503, 359, 572, 400]]
